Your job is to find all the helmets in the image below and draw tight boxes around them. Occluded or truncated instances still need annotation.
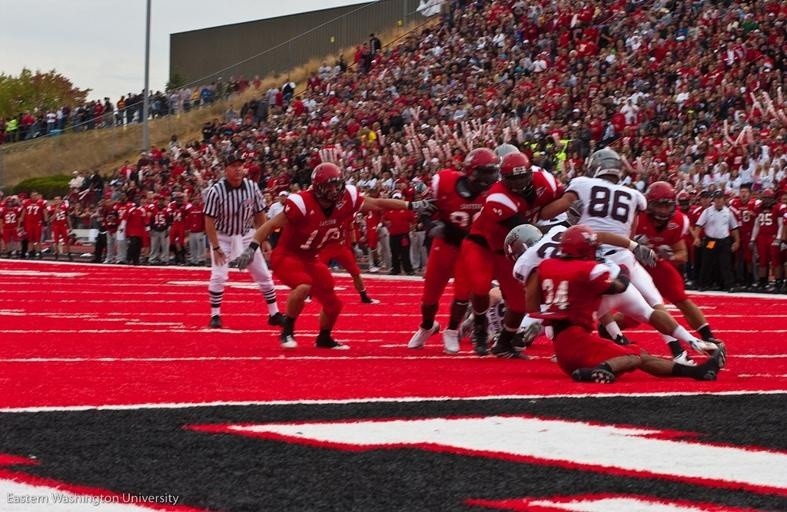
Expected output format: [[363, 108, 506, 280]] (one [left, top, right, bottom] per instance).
[[560, 224, 600, 261], [645, 180, 677, 225], [587, 148, 622, 179], [503, 223, 542, 262], [311, 162, 346, 203], [463, 143, 532, 195]]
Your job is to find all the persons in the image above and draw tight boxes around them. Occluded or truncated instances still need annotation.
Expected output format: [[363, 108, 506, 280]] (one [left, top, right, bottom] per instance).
[[0, 0, 787, 384]]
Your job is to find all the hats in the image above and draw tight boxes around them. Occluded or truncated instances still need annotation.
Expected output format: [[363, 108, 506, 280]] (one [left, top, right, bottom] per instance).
[[224, 154, 247, 168], [678, 188, 775, 206]]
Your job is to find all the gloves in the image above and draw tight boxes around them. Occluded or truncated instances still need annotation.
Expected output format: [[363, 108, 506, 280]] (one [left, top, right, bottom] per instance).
[[631, 243, 658, 268], [563, 200, 584, 225], [771, 239, 781, 247], [656, 245, 676, 261], [747, 241, 754, 250], [405, 197, 438, 217], [237, 247, 255, 269]]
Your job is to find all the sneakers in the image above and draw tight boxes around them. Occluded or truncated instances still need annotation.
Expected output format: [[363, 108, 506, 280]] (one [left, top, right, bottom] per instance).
[[362, 298, 380, 304], [674, 337, 725, 378], [268, 312, 287, 326], [673, 350, 696, 365], [305, 296, 312, 304], [279, 332, 298, 349], [368, 262, 423, 275], [209, 314, 223, 328], [408, 320, 528, 361], [90, 255, 211, 266], [315, 336, 351, 350], [572, 366, 615, 383], [684, 278, 786, 293], [0, 246, 43, 259]]
